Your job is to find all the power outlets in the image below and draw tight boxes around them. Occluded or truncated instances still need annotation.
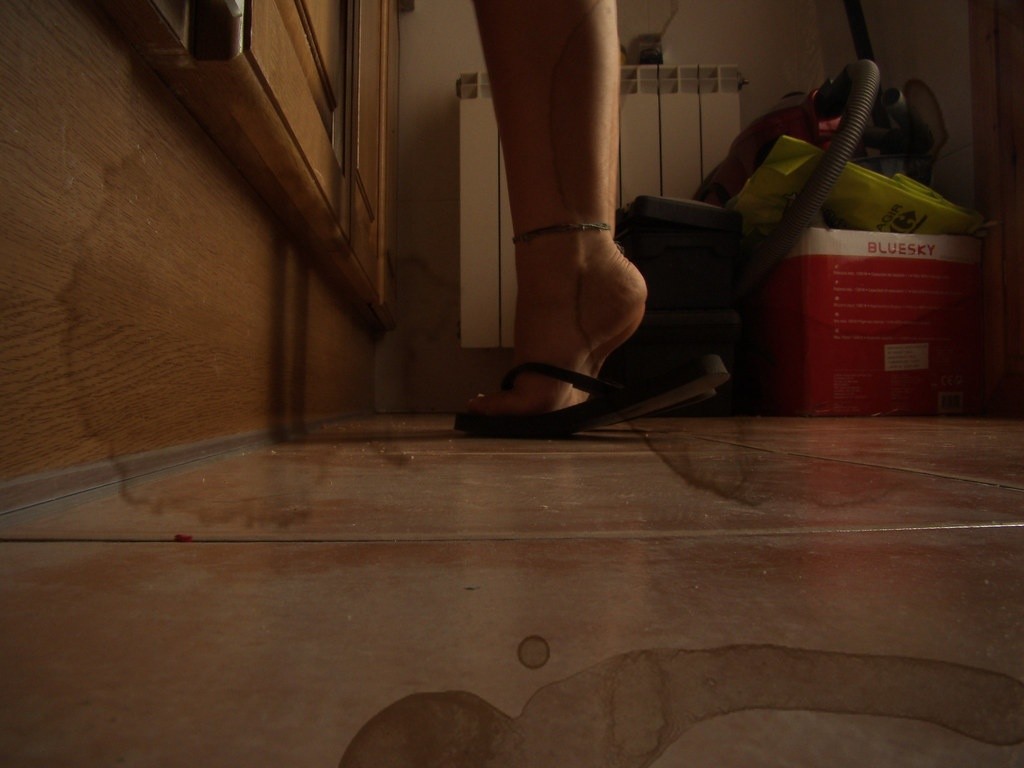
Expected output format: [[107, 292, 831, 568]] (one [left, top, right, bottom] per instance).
[[638, 33, 664, 66]]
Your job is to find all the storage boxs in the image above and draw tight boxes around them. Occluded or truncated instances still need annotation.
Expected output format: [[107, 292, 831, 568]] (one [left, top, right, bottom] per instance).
[[602, 195, 741, 415], [749, 226, 984, 416]]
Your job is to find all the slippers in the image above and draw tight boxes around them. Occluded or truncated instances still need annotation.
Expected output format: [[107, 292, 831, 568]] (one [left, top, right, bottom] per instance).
[[452, 353, 731, 441]]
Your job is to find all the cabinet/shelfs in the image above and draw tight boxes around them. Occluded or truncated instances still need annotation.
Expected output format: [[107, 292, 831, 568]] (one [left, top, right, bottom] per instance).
[[0, 0, 415, 530]]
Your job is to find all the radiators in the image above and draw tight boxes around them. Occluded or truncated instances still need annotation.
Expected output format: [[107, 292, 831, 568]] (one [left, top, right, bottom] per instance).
[[457, 63, 749, 351]]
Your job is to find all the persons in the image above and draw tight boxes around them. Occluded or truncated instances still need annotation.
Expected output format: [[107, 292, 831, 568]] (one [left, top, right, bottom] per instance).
[[452, 0, 729, 438]]
